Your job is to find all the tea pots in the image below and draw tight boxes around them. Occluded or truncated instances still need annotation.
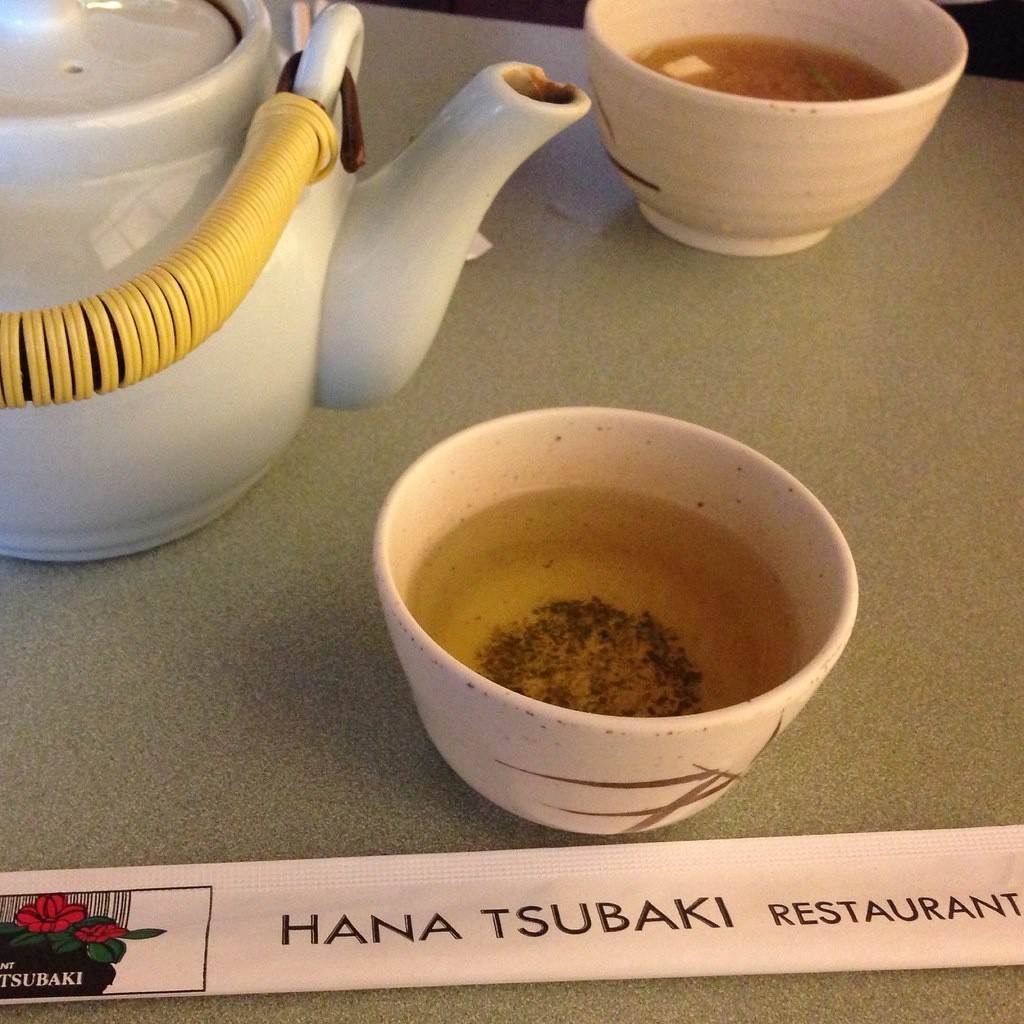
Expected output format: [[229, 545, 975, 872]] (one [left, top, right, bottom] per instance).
[[0, 0, 596, 567]]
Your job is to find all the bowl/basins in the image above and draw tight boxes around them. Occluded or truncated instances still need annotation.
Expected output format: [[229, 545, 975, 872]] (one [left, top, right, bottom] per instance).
[[371, 406, 860, 835], [582, 0, 968, 260]]
